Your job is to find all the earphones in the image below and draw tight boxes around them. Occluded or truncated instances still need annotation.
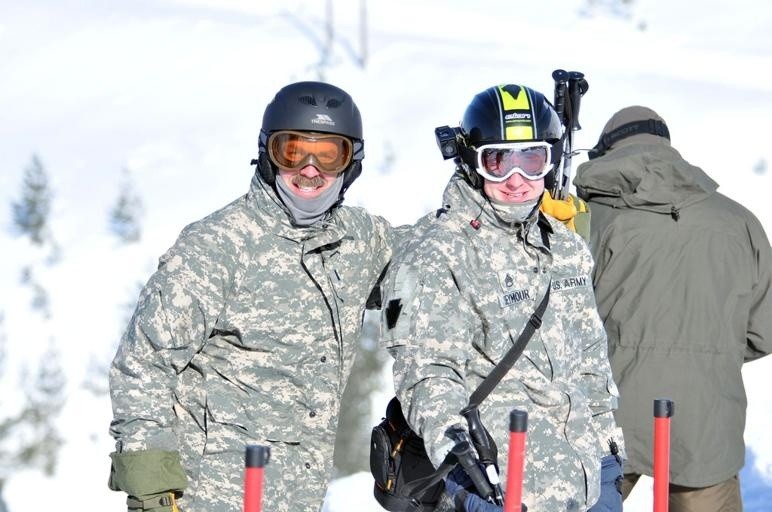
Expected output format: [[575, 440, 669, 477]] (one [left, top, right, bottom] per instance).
[[470, 219, 481, 229]]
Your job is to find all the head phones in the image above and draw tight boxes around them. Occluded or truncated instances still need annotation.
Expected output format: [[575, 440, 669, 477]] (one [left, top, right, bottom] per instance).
[[256, 155, 362, 195], [455, 126, 559, 190]]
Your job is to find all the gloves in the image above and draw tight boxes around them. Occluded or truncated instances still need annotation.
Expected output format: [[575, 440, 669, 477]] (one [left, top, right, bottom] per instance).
[[587, 455, 624, 512], [446, 460, 528, 512], [108, 450, 188, 512]]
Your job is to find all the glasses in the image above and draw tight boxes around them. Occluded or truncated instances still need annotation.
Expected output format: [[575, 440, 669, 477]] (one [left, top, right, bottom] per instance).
[[268, 131, 353, 173], [588, 143, 606, 160], [475, 142, 554, 183]]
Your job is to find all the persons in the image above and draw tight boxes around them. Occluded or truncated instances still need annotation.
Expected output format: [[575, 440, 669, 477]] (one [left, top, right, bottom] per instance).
[[375, 82, 630, 512], [108, 81, 594, 512], [571, 104, 772, 512]]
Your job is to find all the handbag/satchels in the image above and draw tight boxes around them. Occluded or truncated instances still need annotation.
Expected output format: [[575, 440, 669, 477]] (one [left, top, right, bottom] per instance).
[[370, 396, 444, 512]]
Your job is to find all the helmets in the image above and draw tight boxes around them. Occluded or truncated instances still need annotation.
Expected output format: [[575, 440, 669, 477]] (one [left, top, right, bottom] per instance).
[[461, 84, 563, 188], [258, 81, 364, 184], [599, 106, 670, 153]]
